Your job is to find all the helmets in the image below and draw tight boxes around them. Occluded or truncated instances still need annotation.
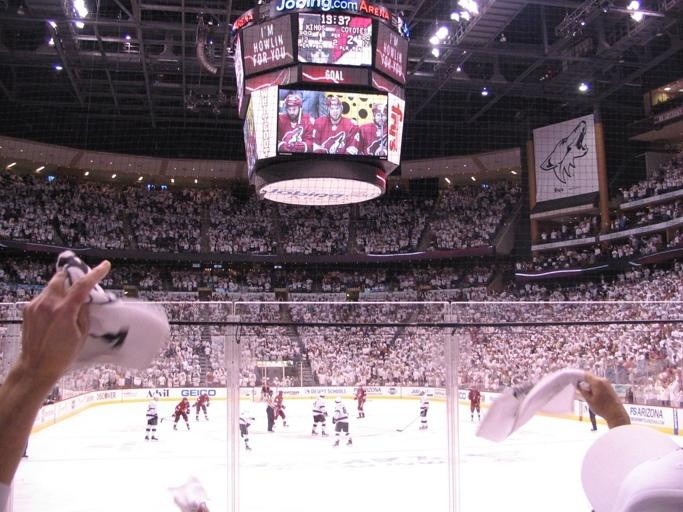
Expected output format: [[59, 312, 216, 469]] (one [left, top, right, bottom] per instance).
[[323, 94, 342, 107], [371, 102, 387, 114], [283, 94, 302, 108]]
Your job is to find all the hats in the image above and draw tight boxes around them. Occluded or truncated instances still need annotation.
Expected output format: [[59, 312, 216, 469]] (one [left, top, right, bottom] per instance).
[[581, 424, 683, 512]]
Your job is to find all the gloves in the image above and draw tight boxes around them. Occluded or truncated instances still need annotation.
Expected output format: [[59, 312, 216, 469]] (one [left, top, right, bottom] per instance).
[[277, 140, 306, 153]]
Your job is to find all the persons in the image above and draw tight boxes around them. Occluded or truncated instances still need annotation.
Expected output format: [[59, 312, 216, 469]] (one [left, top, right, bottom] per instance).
[[272, 390, 290, 427], [144, 404, 158, 441], [310, 393, 329, 436], [0, 151, 683, 414], [465, 385, 481, 423], [311, 95, 358, 154], [170, 396, 191, 432], [352, 384, 367, 419], [262, 390, 277, 433], [278, 88, 326, 120], [237, 414, 255, 450], [0, 254, 114, 512], [418, 390, 430, 430], [331, 396, 352, 447], [570, 368, 682, 512], [191, 393, 211, 421], [353, 102, 389, 154], [277, 93, 316, 154], [585, 398, 599, 433]]
